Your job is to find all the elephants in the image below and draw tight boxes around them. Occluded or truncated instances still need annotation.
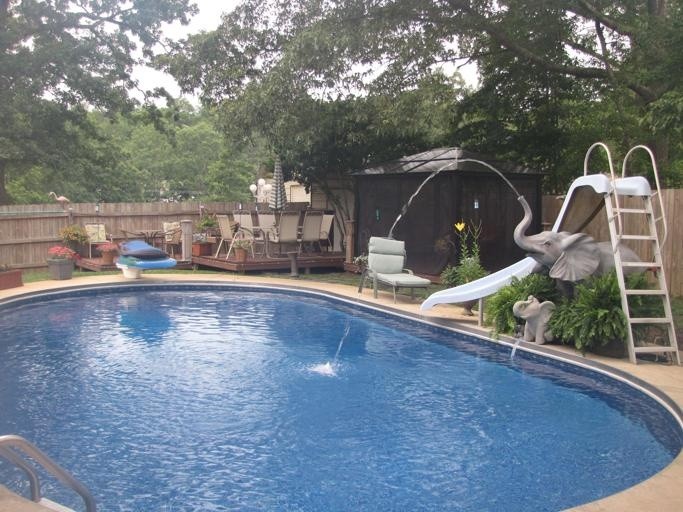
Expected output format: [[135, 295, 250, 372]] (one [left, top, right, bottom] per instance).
[[512, 193, 649, 307], [512, 294, 557, 345]]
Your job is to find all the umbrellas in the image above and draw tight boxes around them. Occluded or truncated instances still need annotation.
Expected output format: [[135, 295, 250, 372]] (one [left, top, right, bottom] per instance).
[[268, 154, 289, 253]]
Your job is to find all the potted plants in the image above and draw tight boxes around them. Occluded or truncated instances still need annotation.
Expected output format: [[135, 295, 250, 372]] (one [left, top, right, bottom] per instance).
[[0, 264, 23, 290], [192, 211, 215, 256], [569, 270, 664, 357], [440, 260, 491, 316], [58, 224, 87, 251]]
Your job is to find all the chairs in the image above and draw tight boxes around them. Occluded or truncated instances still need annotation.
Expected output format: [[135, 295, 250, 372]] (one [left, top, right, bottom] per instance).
[[358, 237, 431, 304], [215, 208, 335, 261], [121, 221, 182, 258], [85, 221, 113, 259]]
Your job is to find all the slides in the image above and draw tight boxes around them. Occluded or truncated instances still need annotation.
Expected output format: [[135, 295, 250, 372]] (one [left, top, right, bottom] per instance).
[[418, 174, 650, 314]]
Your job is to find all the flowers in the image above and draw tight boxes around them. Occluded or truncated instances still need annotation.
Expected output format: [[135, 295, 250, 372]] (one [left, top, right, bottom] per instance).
[[48, 245, 81, 261], [96, 242, 119, 252]]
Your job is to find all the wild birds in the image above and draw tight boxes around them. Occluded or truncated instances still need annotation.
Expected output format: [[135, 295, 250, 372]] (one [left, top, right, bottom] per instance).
[[48, 191, 70, 211]]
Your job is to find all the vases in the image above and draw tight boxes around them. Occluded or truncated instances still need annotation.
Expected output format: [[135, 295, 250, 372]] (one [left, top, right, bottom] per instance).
[[48, 259, 73, 280], [101, 252, 115, 265]]
